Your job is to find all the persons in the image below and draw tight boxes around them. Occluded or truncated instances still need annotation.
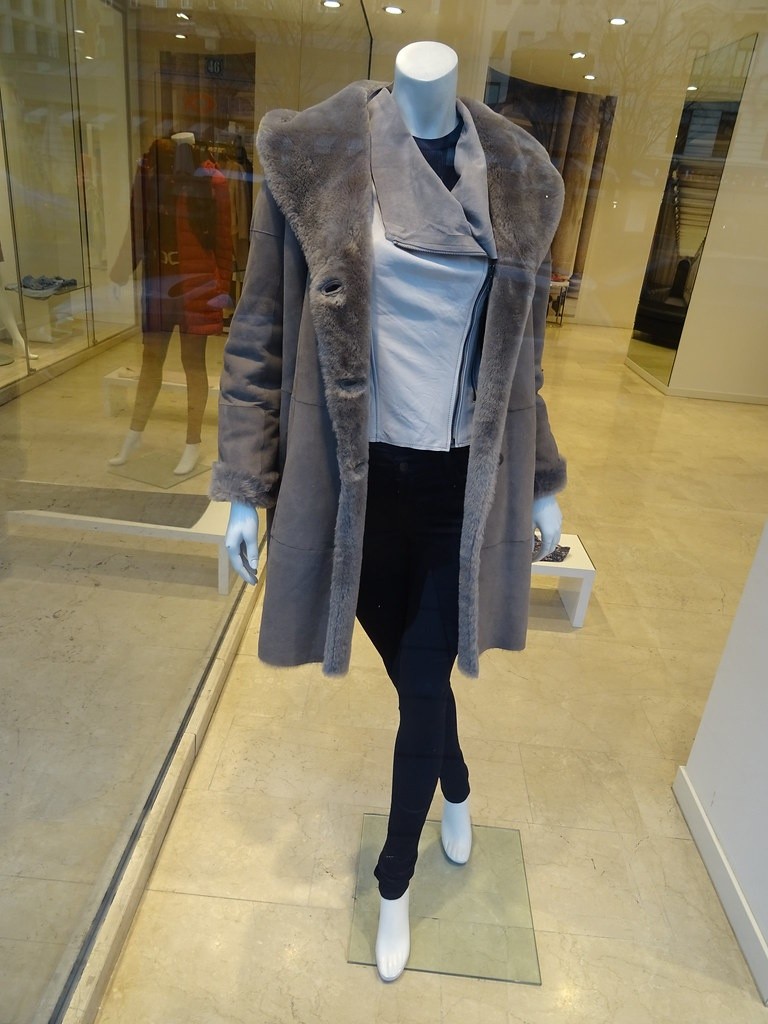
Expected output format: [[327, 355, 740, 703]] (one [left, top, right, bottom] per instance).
[[209, 42, 566, 982], [110, 130, 234, 473], [0, 244, 39, 359]]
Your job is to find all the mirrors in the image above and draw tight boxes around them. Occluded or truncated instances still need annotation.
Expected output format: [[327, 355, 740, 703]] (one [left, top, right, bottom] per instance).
[[626, 32, 759, 387]]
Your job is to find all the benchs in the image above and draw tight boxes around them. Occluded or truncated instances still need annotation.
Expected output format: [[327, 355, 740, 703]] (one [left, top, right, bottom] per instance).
[[105, 368, 221, 421], [0, 477, 233, 594], [530, 531, 597, 629]]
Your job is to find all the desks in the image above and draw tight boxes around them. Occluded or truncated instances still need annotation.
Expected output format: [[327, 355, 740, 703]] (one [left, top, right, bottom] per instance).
[[546, 280, 570, 328]]
